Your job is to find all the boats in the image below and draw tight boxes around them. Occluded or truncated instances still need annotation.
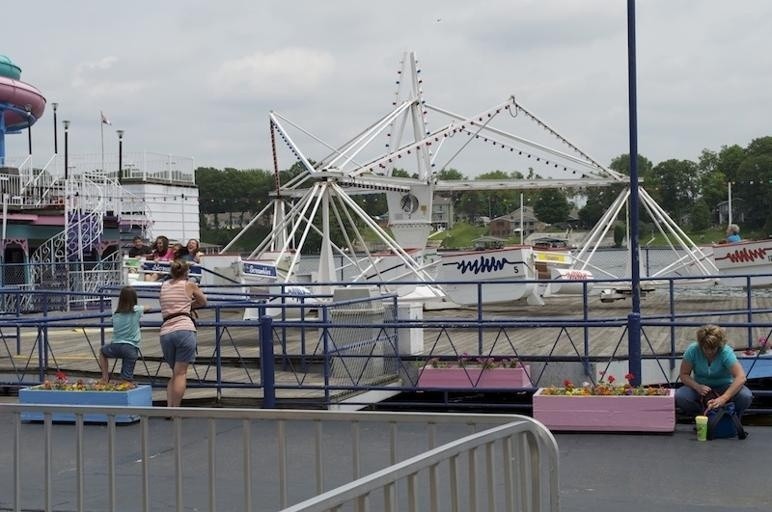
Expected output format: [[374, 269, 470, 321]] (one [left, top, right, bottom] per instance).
[[716, 241, 772, 288], [530, 237, 573, 279], [100, 250, 301, 321]]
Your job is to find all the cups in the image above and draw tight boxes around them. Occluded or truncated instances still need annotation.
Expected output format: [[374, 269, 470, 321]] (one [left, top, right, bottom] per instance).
[[695, 416, 708, 441]]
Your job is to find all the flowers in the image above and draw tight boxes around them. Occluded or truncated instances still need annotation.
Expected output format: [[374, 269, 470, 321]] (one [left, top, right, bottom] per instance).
[[31, 370, 134, 392], [538, 374, 670, 396], [428, 352, 518, 368]]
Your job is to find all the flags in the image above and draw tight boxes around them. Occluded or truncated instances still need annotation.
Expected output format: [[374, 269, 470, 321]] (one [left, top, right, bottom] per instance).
[[102, 114, 111, 126]]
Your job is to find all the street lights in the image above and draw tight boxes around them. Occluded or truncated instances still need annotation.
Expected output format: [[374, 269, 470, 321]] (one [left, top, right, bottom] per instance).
[[116, 128, 124, 184], [63, 119, 70, 178], [52, 102, 58, 154]]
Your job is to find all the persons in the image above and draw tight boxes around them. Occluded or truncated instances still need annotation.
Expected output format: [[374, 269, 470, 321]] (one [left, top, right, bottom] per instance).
[[169, 244, 187, 262], [129, 235, 158, 282], [152, 236, 175, 282], [94, 285, 152, 382], [675, 323, 754, 429], [158, 258, 208, 407], [187, 238, 204, 282]]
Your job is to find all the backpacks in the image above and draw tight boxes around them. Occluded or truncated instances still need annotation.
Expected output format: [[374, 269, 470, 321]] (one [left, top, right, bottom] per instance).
[[698, 384, 749, 441]]
